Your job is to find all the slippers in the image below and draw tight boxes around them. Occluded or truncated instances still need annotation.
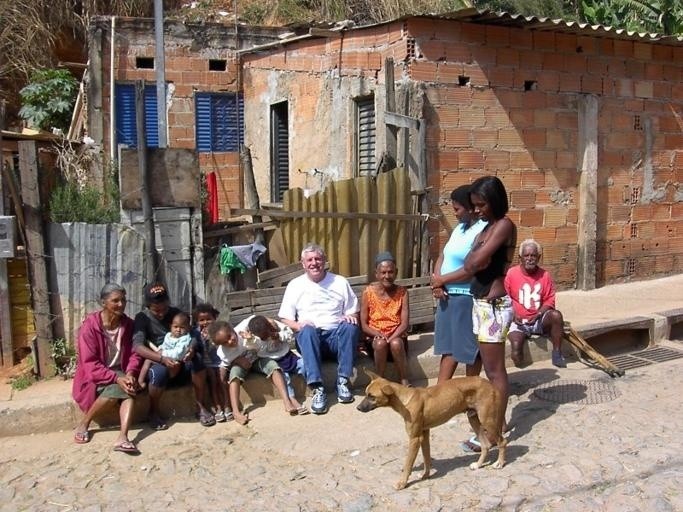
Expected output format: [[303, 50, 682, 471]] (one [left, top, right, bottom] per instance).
[[74, 405, 308, 454], [459, 436, 483, 452]]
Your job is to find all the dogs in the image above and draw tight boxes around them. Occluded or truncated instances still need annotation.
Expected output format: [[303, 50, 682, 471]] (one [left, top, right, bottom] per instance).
[[356, 367, 508, 491]]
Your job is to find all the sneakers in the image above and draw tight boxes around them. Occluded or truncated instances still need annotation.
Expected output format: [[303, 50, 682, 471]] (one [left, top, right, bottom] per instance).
[[310, 385, 328, 413], [335, 376, 353, 402]]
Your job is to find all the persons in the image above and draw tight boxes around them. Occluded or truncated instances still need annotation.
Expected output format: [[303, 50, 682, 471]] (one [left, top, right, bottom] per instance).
[[209, 314, 297, 424], [460, 176, 515, 452], [278, 244, 360, 413], [72, 283, 143, 453], [504, 240, 566, 367], [135, 312, 192, 391], [193, 303, 234, 422], [359, 252, 410, 385], [238, 316, 311, 415], [429, 185, 489, 384], [131, 282, 216, 431]]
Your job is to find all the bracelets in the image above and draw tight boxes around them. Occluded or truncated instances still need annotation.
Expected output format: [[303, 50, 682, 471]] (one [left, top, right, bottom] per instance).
[[159, 355, 163, 364]]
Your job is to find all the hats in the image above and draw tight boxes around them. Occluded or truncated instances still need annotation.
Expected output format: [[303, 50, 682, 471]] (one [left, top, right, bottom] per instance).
[[144, 278, 170, 303], [374, 251, 396, 268]]
